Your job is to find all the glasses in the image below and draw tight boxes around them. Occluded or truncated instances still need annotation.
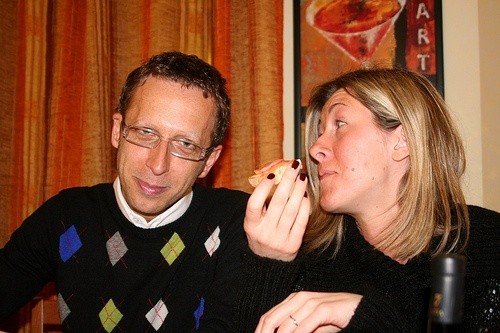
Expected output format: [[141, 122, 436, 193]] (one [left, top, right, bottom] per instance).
[[120, 122, 214, 162]]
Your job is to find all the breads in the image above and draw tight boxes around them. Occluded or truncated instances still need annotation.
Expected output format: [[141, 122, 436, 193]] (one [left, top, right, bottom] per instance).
[[247, 158, 302, 188]]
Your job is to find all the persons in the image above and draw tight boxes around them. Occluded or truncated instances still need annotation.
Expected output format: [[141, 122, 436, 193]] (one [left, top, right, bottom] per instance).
[[1, 50, 270, 333], [231, 65, 500, 333]]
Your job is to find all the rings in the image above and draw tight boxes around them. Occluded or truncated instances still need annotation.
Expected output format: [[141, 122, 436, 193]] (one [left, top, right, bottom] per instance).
[[289, 315, 299, 326]]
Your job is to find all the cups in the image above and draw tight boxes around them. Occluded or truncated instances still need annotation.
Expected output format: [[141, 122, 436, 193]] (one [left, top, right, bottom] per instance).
[[428, 254, 463, 333]]
